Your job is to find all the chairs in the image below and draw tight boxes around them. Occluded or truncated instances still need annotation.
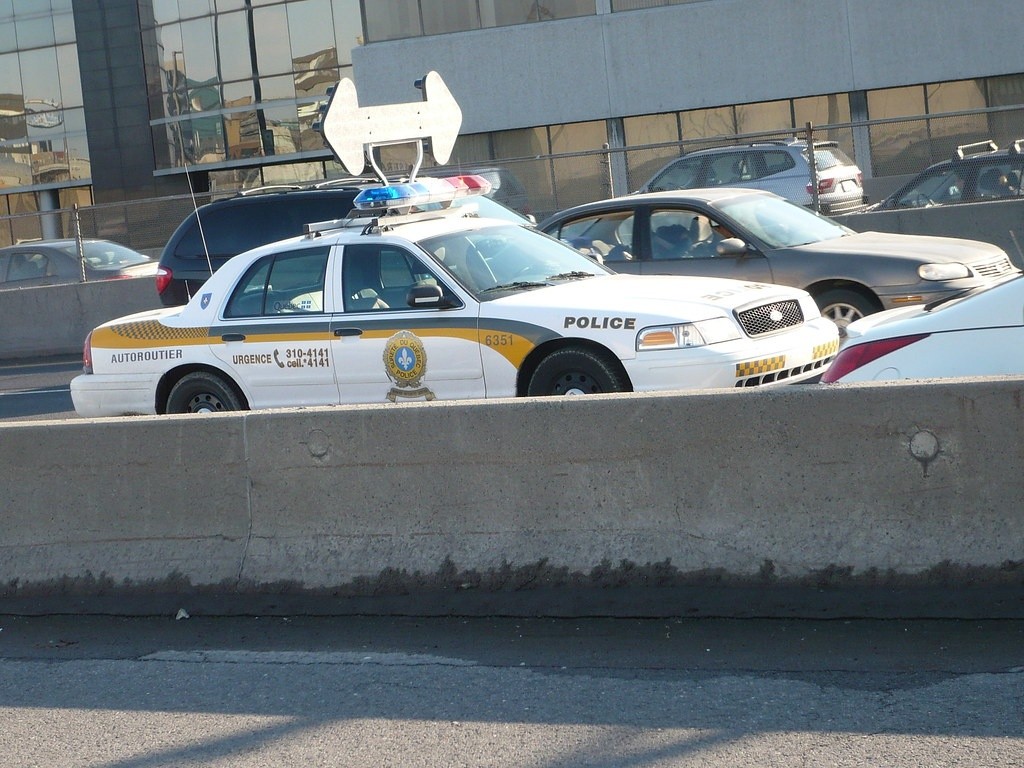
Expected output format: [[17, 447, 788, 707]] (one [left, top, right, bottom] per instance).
[[1007, 170, 1024, 190], [688, 215, 713, 255], [401, 262, 443, 303], [729, 159, 752, 183], [349, 263, 390, 309]]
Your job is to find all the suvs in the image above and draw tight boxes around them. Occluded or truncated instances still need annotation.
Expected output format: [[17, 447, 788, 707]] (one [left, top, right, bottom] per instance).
[[156, 178, 538, 308], [624, 137, 865, 215], [843, 139, 1024, 217]]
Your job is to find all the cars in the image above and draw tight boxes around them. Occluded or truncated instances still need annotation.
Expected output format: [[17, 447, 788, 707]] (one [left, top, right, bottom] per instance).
[[0, 238, 159, 290], [533, 189, 1024, 327], [820, 272, 1024, 385], [68, 175, 841, 415]]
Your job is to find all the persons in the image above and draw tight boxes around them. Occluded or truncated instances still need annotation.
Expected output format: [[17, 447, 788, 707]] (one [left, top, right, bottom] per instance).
[[945, 171, 975, 202], [709, 215, 738, 252]]
